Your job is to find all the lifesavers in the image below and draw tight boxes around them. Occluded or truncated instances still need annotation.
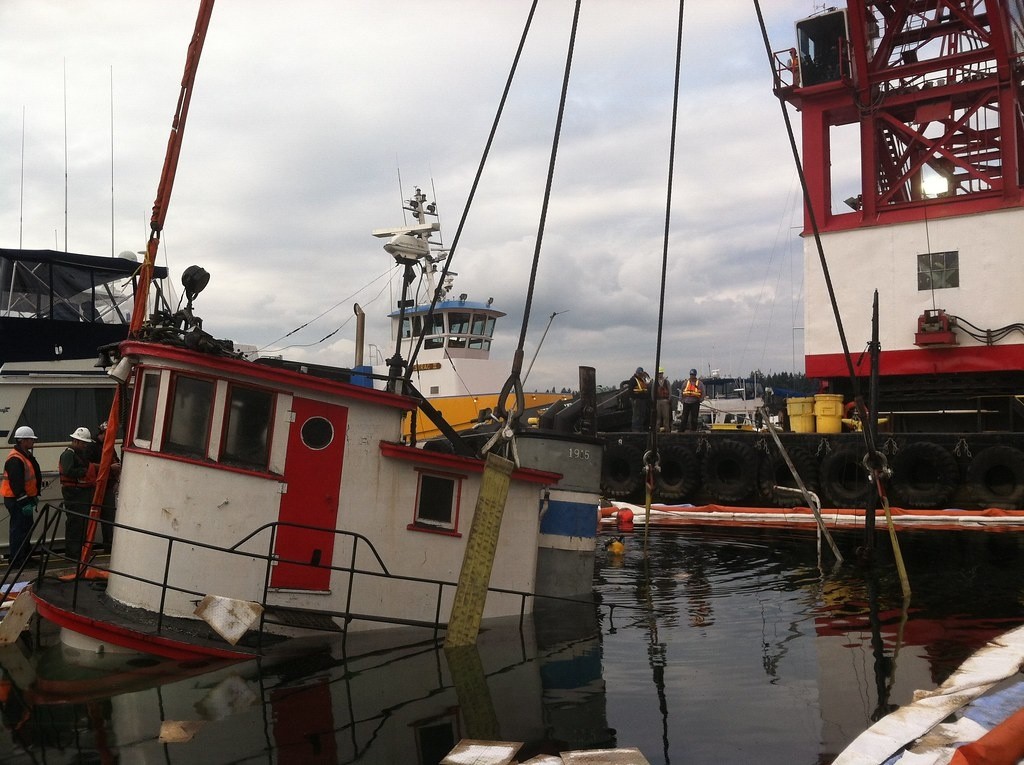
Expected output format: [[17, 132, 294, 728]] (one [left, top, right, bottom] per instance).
[[594, 438, 1024, 508]]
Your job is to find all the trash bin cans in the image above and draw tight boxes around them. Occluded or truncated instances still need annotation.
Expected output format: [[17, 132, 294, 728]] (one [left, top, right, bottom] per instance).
[[787, 396, 816, 432], [814, 394, 844, 433]]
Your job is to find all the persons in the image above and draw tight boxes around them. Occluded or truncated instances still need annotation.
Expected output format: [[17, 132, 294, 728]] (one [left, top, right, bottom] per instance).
[[628, 367, 650, 432], [86, 421, 122, 553], [787, 49, 800, 85], [651, 366, 671, 433], [59, 427, 97, 558], [1, 426, 42, 568], [678, 369, 705, 433]]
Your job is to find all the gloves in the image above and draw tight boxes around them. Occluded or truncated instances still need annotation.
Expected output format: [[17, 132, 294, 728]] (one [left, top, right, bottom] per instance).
[[22, 504, 33, 517], [30, 497, 39, 507]]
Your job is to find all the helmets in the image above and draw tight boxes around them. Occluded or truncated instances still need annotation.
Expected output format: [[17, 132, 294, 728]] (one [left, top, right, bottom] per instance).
[[690, 369, 697, 376], [14, 426, 38, 439], [659, 368, 664, 373], [69, 427, 92, 443], [636, 367, 643, 374]]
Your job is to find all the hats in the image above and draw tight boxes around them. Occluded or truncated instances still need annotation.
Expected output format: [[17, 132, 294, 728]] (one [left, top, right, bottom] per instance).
[[98, 421, 107, 431]]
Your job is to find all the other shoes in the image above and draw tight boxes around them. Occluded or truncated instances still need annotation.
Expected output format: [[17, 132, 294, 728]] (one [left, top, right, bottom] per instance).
[[691, 429, 696, 432], [65, 550, 81, 560], [10, 558, 40, 569], [678, 430, 685, 432]]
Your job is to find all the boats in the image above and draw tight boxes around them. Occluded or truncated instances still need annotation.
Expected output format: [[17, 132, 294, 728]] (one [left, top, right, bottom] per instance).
[[375, 294, 575, 450], [0, 265, 601, 695], [0, 352, 138, 628], [670, 375, 783, 432], [596, 0, 1024, 537]]
